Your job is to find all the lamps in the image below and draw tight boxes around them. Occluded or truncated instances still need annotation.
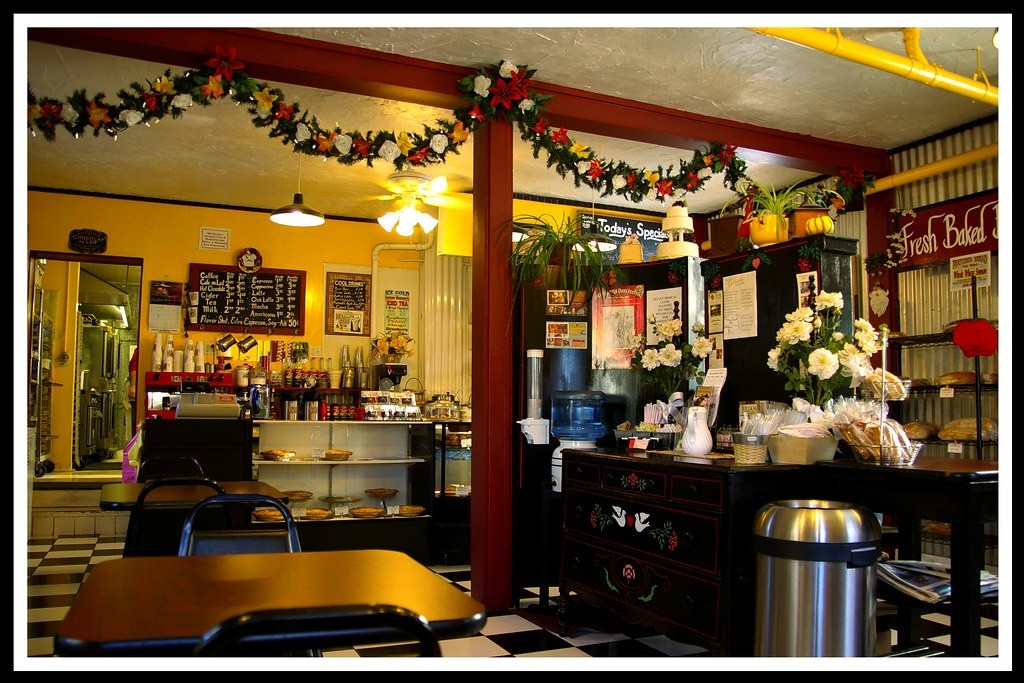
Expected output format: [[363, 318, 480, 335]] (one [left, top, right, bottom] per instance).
[[269, 151, 325, 227], [376, 196, 438, 236]]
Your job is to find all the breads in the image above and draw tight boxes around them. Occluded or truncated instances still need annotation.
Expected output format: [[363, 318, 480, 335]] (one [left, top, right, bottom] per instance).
[[445, 485, 457, 495], [925, 521, 951, 536], [838, 370, 993, 459]]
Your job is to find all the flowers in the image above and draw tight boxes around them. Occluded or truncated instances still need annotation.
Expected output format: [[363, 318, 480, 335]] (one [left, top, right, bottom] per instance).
[[627, 313, 713, 397], [369, 331, 415, 361], [28, 45, 877, 206], [767, 290, 879, 407], [864, 208, 918, 277]]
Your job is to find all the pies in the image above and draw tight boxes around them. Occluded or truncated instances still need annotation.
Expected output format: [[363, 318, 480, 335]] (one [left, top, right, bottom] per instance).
[[254, 488, 426, 520], [324, 449, 354, 461], [260, 449, 296, 461]]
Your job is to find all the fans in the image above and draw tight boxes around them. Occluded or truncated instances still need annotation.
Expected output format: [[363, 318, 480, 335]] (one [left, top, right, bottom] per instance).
[[341, 162, 472, 207]]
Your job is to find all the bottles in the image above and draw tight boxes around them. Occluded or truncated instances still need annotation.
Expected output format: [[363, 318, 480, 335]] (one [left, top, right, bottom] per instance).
[[323, 393, 355, 421], [551, 390, 606, 442], [284, 356, 333, 389], [460, 404, 469, 422]]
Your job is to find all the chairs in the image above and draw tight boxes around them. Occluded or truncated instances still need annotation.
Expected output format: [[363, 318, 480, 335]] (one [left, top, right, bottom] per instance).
[[193, 603, 444, 657], [123, 477, 226, 558], [178, 494, 323, 657], [136, 454, 204, 483]]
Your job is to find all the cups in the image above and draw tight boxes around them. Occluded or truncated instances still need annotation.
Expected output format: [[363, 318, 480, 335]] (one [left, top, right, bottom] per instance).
[[237, 335, 258, 353], [151, 333, 205, 373], [189, 292, 198, 305], [216, 333, 237, 352], [328, 370, 342, 389], [237, 368, 249, 387], [188, 307, 197, 324]]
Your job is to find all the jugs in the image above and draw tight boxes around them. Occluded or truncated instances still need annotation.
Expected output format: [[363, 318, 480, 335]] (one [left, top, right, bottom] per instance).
[[420, 392, 460, 420], [681, 406, 713, 455]]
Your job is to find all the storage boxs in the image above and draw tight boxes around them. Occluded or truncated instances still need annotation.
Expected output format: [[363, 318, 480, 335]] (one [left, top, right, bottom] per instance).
[[767, 434, 839, 465]]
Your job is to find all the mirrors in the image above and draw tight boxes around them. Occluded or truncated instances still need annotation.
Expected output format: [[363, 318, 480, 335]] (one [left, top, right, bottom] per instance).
[[28, 248, 144, 491]]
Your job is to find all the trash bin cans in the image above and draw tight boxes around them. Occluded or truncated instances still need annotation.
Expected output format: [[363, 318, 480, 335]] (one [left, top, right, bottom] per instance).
[[749, 500, 884, 657]]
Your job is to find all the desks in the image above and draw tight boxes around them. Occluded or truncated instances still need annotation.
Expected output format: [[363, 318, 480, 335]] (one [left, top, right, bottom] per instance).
[[99, 480, 289, 511], [53, 546, 487, 657]]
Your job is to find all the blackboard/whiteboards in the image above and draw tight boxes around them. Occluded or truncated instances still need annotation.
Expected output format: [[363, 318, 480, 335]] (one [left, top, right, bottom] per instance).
[[186, 263, 307, 336], [577, 209, 694, 265], [67, 229, 108, 253], [333, 279, 366, 310]]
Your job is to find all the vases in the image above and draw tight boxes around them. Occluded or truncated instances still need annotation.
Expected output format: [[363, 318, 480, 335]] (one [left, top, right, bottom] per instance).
[[379, 354, 403, 363]]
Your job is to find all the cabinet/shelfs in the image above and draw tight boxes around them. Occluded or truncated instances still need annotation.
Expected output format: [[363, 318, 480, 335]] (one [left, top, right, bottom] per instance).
[[855, 277, 998, 461], [411, 421, 472, 566], [252, 419, 433, 567], [815, 456, 998, 657], [557, 448, 816, 657], [32, 308, 55, 477]]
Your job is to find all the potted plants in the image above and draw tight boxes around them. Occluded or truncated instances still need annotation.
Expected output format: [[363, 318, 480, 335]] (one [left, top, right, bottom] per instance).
[[490, 214, 629, 313], [719, 181, 845, 248]]
